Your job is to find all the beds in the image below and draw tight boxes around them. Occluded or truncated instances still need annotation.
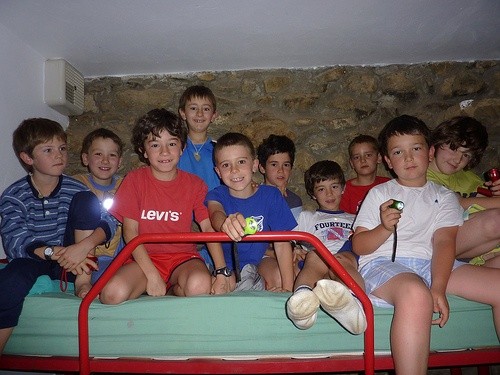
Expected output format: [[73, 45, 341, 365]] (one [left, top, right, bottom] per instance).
[[0, 231, 500, 375]]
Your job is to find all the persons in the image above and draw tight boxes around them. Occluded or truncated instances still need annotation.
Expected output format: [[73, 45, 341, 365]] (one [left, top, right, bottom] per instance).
[[352, 113, 500, 375], [0, 85, 500, 353]]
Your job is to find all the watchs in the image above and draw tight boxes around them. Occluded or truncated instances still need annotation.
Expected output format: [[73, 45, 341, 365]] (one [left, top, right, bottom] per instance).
[[43, 246, 55, 260], [211, 266, 232, 277]]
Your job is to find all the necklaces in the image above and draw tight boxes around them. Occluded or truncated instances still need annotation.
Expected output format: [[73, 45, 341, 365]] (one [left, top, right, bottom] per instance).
[[187, 133, 207, 162]]
